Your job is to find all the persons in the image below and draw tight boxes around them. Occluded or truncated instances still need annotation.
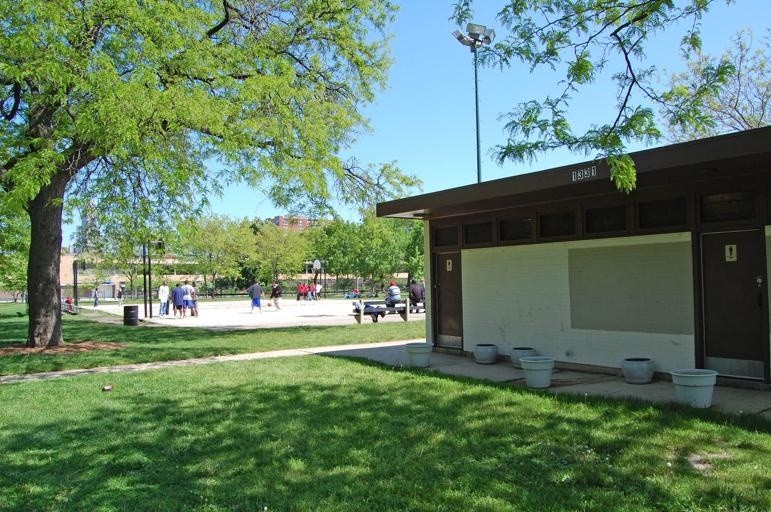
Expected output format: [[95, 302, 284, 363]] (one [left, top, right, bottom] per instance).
[[247, 280, 264, 313], [117, 288, 122, 307], [384, 281, 401, 315], [296, 282, 323, 301], [191, 281, 200, 317], [409, 279, 424, 314], [172, 283, 186, 319], [181, 280, 195, 319], [158, 280, 170, 318], [94, 287, 99, 308], [269, 281, 283, 312]]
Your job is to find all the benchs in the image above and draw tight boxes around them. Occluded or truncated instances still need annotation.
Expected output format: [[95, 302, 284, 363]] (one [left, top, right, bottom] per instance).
[[349, 298, 426, 325]]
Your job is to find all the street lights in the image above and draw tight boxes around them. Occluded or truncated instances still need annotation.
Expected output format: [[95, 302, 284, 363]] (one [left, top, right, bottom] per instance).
[[450, 22, 496, 183]]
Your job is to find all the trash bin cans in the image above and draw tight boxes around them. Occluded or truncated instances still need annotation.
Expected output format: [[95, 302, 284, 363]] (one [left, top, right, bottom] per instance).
[[124, 306, 138, 326]]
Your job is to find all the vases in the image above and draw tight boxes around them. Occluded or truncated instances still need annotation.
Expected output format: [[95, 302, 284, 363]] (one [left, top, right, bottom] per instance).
[[519, 356, 556, 388], [622, 357, 654, 384], [670, 368, 718, 408], [511, 347, 536, 369], [405, 343, 434, 368], [473, 344, 498, 365]]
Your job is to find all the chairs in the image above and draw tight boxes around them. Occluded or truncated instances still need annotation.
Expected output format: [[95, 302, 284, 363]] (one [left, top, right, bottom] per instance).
[[61, 301, 82, 314]]
